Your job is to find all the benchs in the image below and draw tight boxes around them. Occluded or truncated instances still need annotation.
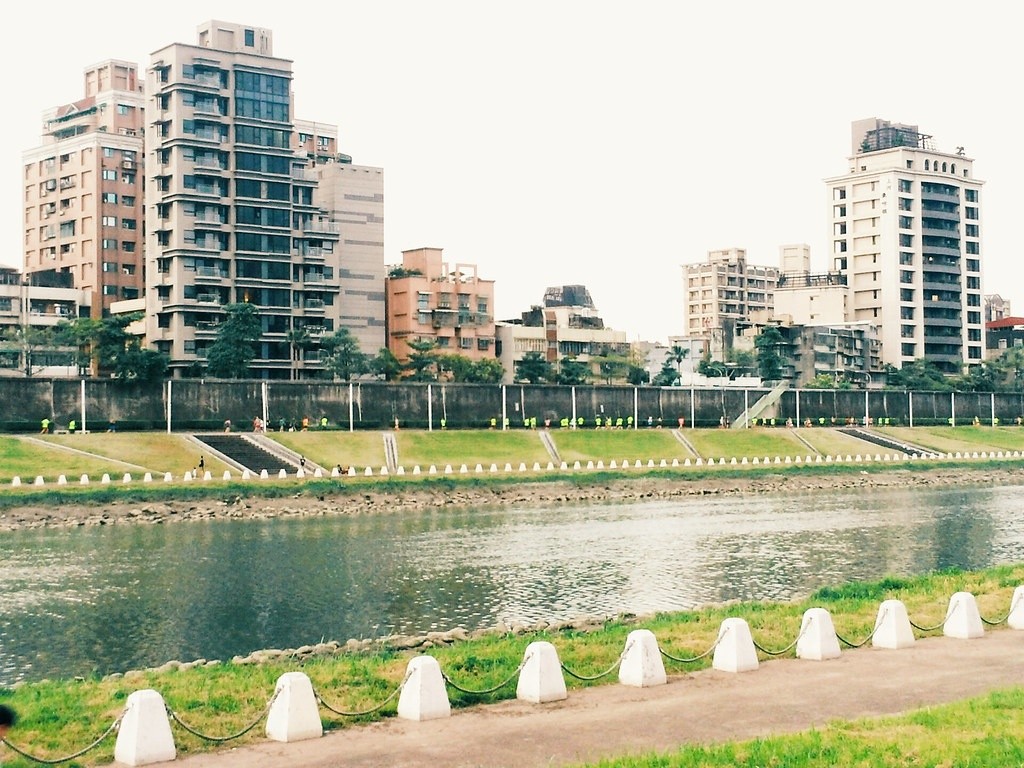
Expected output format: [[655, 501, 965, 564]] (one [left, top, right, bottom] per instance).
[[74, 430, 90, 434], [267, 429, 274, 432], [53, 429, 70, 434]]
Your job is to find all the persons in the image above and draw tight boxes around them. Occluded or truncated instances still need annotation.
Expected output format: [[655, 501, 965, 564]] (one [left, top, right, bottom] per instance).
[[253, 416, 261, 433], [192, 467, 197, 479], [225, 417, 231, 433], [490, 417, 496, 429], [321, 416, 327, 429], [199, 456, 206, 472], [69, 419, 76, 434], [524, 417, 551, 431], [279, 417, 286, 432], [298, 455, 306, 473], [506, 417, 509, 429], [394, 416, 399, 431], [440, 418, 446, 429], [106, 416, 118, 433], [560, 416, 890, 429], [301, 416, 309, 431], [948, 416, 1022, 427], [336, 463, 350, 475], [40, 417, 50, 434], [290, 417, 297, 431]]
